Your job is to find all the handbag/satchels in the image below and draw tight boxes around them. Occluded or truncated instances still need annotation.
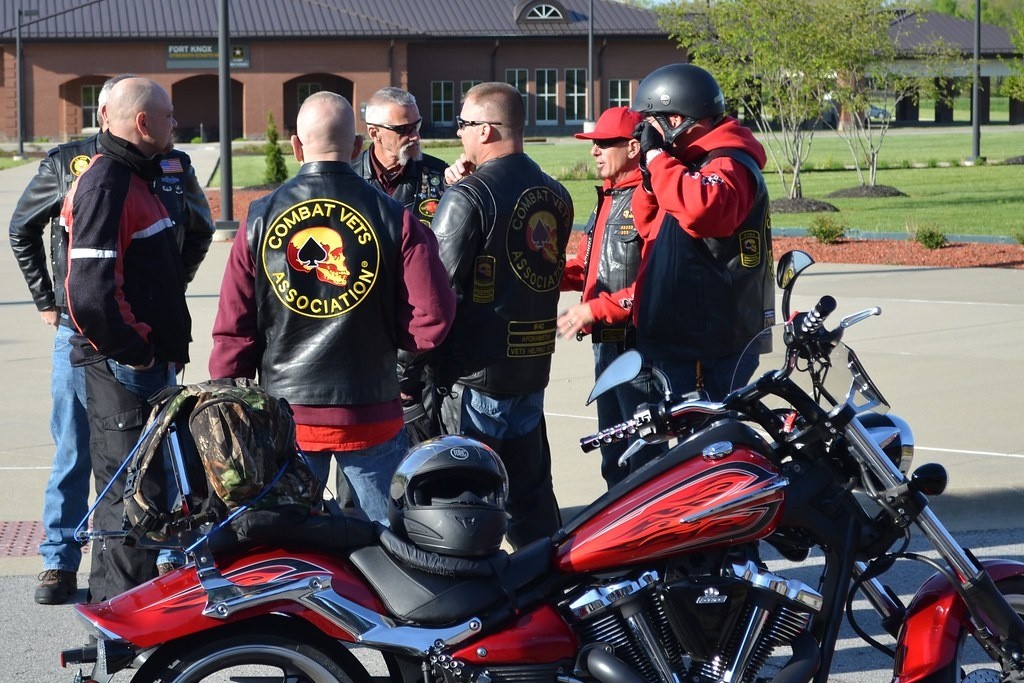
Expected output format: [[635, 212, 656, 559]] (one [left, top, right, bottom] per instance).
[[112, 377, 324, 544]]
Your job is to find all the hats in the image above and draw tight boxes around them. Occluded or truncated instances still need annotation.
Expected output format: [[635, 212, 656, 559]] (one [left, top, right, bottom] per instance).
[[575, 106, 643, 140]]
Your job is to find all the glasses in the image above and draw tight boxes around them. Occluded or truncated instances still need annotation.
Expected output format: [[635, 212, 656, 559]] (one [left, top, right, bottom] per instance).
[[365, 117, 422, 135], [456, 116, 502, 130]]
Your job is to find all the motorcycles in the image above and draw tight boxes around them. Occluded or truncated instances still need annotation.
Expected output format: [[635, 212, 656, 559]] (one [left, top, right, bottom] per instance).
[[62, 250, 1024, 683]]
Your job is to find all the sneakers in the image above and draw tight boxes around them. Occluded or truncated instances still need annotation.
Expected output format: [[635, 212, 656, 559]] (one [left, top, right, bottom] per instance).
[[34, 569, 77, 604]]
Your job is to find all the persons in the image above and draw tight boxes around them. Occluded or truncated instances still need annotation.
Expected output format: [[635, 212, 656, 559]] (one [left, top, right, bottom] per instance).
[[10, 74, 215, 604], [556, 63, 776, 490], [209, 82, 574, 550]]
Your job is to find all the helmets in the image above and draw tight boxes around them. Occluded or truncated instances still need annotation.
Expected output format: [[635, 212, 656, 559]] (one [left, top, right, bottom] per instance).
[[386, 434, 510, 558], [628, 63, 725, 120]]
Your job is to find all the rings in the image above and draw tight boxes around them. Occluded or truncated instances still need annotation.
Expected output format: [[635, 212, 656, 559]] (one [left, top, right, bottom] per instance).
[[568, 322, 572, 326]]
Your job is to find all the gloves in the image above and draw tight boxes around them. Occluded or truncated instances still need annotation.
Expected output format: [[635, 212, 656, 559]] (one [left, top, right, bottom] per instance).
[[631, 121, 664, 157]]
